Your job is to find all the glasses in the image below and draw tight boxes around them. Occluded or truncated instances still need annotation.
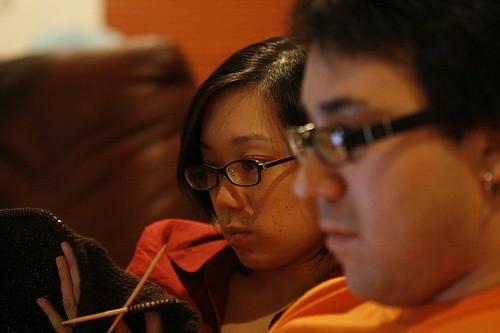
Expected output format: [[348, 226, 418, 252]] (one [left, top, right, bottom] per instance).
[[184, 153, 295, 191], [285, 105, 433, 168]]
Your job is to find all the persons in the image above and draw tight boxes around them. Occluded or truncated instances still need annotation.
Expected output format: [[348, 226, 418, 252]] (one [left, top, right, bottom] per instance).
[[37, 32, 342, 333], [270, 0, 500, 333]]
